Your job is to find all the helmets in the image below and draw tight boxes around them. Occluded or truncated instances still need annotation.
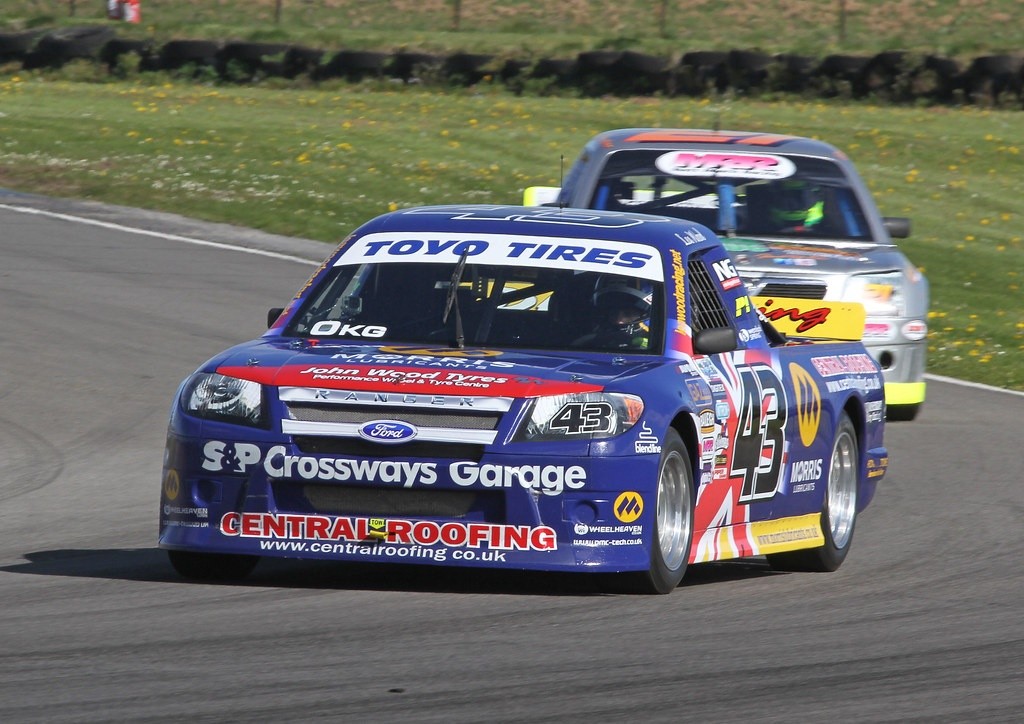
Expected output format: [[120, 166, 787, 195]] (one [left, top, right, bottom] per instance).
[[594, 274, 654, 335], [764, 182, 823, 227]]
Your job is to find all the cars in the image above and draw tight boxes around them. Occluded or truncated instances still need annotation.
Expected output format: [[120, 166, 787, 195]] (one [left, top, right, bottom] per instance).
[[524, 128, 930, 422], [158, 204, 888, 595]]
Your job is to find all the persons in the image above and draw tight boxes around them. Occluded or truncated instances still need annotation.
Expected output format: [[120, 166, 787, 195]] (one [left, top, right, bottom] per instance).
[[570, 273, 655, 353], [758, 173, 839, 240]]
